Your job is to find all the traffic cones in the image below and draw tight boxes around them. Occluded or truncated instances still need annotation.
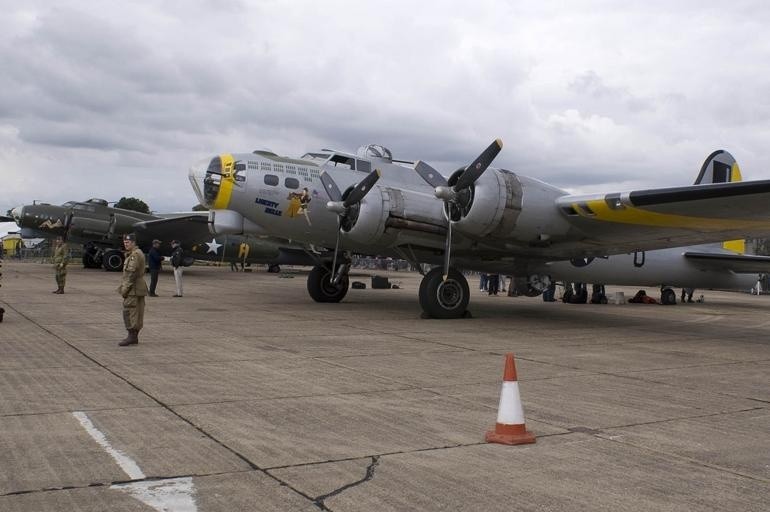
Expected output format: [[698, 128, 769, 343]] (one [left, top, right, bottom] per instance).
[[484, 350, 537, 446]]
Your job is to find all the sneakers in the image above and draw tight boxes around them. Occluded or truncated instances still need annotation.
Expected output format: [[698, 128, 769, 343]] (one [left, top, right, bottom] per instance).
[[149, 290, 158, 297], [480, 289, 558, 302], [172, 295, 183, 297]]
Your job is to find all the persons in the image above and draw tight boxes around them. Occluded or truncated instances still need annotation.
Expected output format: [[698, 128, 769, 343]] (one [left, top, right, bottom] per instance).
[[473, 268, 704, 303], [171, 240, 184, 297], [118, 234, 148, 346], [290, 188, 312, 227], [15, 239, 22, 260], [52, 236, 68, 293], [350, 252, 431, 275], [149, 240, 164, 297], [228, 243, 245, 272]]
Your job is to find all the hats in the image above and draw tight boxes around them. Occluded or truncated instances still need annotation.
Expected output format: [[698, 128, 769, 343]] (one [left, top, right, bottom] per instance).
[[123, 234, 136, 240], [170, 240, 179, 244], [152, 239, 162, 244]]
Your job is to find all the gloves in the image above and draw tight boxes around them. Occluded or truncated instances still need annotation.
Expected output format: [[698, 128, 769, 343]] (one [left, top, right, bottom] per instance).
[[60, 263, 64, 267], [121, 287, 130, 298]]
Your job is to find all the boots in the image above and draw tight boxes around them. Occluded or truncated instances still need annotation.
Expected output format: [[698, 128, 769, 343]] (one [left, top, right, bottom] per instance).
[[118, 329, 139, 346], [52, 286, 64, 294]]
